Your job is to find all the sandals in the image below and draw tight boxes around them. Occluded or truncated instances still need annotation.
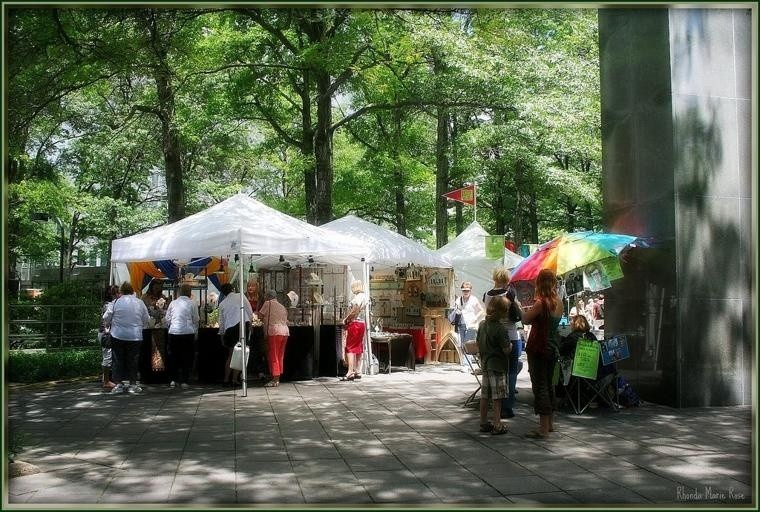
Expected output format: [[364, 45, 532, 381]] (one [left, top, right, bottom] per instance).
[[340, 371, 361, 381], [480, 422, 507, 434]]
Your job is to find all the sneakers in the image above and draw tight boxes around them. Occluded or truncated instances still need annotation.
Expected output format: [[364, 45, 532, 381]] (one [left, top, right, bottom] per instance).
[[112, 383, 142, 393]]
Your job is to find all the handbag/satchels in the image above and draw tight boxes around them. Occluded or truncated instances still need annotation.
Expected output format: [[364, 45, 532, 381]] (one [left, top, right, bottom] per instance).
[[101, 333, 112, 349], [256, 337, 269, 353], [527, 317, 547, 353]]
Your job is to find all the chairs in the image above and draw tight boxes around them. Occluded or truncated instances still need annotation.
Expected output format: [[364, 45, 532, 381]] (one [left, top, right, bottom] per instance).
[[555, 344, 627, 417], [460, 337, 522, 408]]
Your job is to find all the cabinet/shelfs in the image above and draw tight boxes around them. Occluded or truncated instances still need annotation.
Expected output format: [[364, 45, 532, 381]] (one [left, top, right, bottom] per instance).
[[422, 308, 453, 363]]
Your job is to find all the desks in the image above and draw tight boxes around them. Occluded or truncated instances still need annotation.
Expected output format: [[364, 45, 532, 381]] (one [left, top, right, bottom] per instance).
[[362, 329, 417, 375], [111, 316, 340, 386]]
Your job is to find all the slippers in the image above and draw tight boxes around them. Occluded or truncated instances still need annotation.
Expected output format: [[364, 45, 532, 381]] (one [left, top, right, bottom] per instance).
[[526, 430, 545, 438]]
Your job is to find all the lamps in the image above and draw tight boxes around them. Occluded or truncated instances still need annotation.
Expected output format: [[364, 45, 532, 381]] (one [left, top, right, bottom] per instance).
[[210, 253, 318, 278]]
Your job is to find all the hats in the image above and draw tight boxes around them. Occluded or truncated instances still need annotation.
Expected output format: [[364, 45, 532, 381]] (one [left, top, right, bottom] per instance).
[[461, 282, 472, 291]]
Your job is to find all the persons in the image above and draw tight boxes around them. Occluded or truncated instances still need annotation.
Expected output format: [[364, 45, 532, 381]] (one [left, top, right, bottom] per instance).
[[480, 266, 522, 419], [165, 283, 201, 390], [242, 278, 260, 312], [339, 279, 367, 382], [218, 283, 255, 388], [569, 292, 606, 332], [258, 289, 292, 387], [102, 281, 152, 394], [475, 295, 514, 434], [563, 313, 596, 356], [516, 266, 563, 439], [97, 284, 120, 390], [140, 278, 167, 309], [448, 280, 486, 372]]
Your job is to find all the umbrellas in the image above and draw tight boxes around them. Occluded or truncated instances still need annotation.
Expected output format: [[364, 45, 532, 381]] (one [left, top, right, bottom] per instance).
[[504, 229, 654, 286]]
